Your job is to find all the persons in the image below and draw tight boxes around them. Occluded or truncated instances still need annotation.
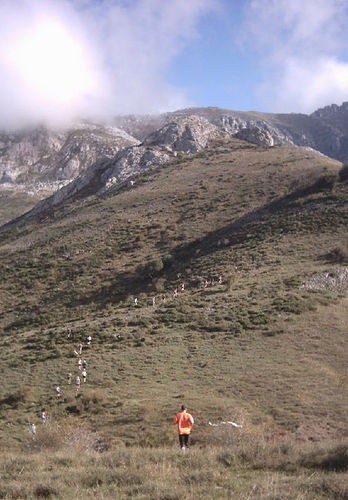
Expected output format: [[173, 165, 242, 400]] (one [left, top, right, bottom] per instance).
[[32, 335, 93, 440], [174, 405, 195, 453]]
